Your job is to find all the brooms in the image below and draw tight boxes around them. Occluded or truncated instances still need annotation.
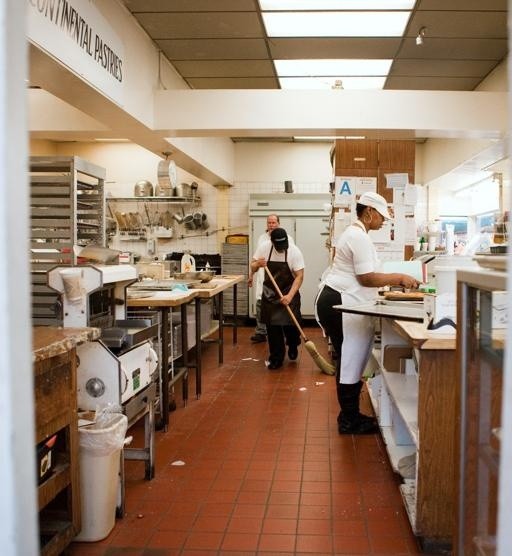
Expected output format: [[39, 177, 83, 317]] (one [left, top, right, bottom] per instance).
[[263, 265, 336, 376]]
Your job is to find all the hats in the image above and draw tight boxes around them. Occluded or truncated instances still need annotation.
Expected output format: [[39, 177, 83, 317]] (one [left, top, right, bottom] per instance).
[[356, 191, 394, 220], [270, 228, 289, 251]]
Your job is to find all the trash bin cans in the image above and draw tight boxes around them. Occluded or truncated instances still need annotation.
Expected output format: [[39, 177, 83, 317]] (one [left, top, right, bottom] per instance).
[[72, 412, 126, 542]]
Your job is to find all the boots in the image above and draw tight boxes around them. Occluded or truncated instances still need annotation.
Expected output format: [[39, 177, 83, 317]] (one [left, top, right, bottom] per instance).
[[336, 381, 378, 435]]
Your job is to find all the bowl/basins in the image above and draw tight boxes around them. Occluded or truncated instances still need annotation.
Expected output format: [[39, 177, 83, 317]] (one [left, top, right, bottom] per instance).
[[174, 183, 193, 197], [155, 184, 175, 198], [185, 270, 217, 283], [134, 180, 153, 197]]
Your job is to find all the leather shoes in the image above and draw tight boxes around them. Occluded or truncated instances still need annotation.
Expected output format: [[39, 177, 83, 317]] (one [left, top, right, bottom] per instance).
[[267, 357, 283, 370], [250, 334, 266, 342], [288, 345, 298, 360]]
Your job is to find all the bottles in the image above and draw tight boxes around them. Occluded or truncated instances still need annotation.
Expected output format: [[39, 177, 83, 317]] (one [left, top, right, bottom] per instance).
[[205, 262, 211, 273], [419, 238, 426, 250]]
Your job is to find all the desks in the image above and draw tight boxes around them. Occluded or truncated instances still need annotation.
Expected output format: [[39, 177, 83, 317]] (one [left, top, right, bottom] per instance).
[[31, 325, 102, 556]]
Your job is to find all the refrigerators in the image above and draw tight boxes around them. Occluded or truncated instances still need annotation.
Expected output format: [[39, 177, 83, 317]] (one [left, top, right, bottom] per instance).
[[248, 192, 335, 320]]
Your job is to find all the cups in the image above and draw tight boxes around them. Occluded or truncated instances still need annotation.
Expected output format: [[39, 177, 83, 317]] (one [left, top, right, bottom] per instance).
[[170, 211, 211, 230], [422, 243, 429, 252], [428, 236, 437, 252], [59, 267, 83, 305], [413, 243, 422, 252]]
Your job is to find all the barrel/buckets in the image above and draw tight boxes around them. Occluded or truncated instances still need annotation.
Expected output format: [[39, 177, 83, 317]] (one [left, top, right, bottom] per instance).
[[181, 252, 196, 273]]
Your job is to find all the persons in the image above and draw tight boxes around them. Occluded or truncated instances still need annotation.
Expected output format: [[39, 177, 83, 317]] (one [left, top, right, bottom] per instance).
[[246, 214, 296, 342], [312, 191, 422, 435], [250, 227, 305, 370]]
